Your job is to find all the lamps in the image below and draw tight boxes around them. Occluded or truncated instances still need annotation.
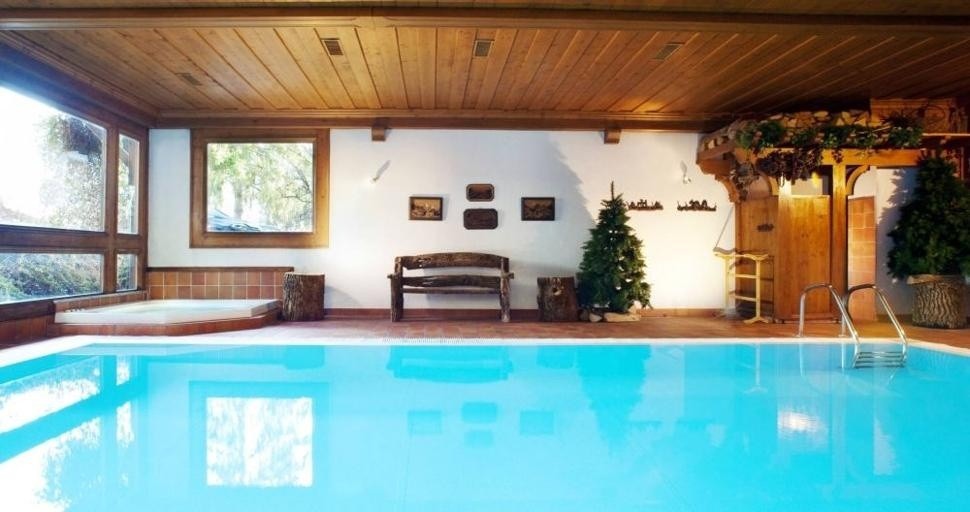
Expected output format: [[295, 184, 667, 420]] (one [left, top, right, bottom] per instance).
[[370, 125, 387, 141], [602, 126, 622, 144]]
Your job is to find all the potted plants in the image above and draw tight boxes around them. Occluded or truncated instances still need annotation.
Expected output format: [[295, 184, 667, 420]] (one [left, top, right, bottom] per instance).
[[883, 146, 970, 328]]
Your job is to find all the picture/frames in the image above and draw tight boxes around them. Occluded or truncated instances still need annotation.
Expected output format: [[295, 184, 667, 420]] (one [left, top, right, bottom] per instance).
[[408, 183, 556, 230]]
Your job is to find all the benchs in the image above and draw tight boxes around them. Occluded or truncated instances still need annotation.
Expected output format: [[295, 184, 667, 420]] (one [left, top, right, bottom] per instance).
[[386, 250, 514, 324]]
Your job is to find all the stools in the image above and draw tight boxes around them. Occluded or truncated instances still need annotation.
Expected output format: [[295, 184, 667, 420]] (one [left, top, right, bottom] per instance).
[[281, 271, 326, 321], [536, 276, 579, 322]]
